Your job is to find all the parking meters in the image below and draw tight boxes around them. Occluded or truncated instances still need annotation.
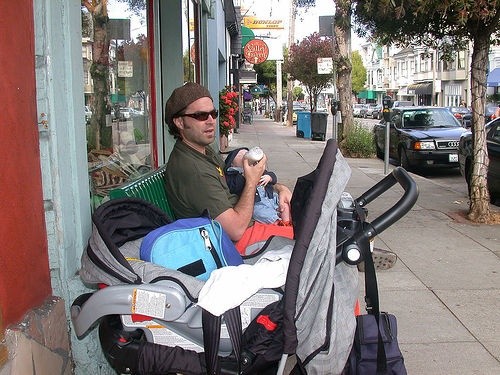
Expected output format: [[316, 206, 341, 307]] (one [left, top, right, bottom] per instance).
[[330, 99, 338, 139], [382, 94, 394, 175]]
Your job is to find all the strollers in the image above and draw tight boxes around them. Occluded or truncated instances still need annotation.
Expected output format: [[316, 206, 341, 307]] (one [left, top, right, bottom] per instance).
[[68, 137, 422, 373]]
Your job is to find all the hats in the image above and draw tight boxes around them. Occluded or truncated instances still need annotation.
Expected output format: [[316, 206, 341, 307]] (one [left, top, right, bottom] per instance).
[[165, 82, 213, 125], [226, 148, 249, 171]]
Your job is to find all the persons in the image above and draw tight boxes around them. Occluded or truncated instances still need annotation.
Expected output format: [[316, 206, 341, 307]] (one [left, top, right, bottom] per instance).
[[165, 83, 398, 273], [224, 147, 290, 226]]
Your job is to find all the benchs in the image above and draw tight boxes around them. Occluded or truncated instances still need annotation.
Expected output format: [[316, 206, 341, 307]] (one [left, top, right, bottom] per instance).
[[109, 162, 177, 224], [241, 112, 251, 125]]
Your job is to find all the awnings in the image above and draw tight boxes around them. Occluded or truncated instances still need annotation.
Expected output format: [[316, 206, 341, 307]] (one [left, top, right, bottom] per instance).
[[396, 83, 432, 97], [486, 68, 500, 88]]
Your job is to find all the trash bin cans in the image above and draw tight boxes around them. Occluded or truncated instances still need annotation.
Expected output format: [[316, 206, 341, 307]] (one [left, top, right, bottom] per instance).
[[295, 111, 311, 138], [311, 112, 329, 141]]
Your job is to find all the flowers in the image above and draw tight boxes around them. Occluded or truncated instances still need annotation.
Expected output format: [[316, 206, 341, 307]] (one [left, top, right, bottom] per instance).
[[218, 85, 238, 139]]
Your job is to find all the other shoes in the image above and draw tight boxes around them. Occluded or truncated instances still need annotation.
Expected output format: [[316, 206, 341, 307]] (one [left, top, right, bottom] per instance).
[[358, 247, 397, 272]]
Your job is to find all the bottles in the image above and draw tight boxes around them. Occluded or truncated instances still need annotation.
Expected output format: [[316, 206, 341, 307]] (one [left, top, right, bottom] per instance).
[[243, 147, 264, 166]]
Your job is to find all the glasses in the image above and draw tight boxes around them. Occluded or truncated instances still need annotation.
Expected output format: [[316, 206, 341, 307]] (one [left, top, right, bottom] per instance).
[[178, 109, 218, 123]]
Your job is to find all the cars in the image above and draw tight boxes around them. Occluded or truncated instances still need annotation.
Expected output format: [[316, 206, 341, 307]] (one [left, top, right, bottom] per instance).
[[445, 106, 472, 127], [392, 101, 414, 108], [373, 105, 470, 177], [458, 117, 500, 204], [292, 102, 381, 120], [462, 106, 498, 128], [84, 104, 144, 124]]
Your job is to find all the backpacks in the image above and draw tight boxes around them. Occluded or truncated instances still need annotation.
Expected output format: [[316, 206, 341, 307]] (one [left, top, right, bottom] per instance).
[[139, 216, 245, 285], [341, 313, 407, 375]]
